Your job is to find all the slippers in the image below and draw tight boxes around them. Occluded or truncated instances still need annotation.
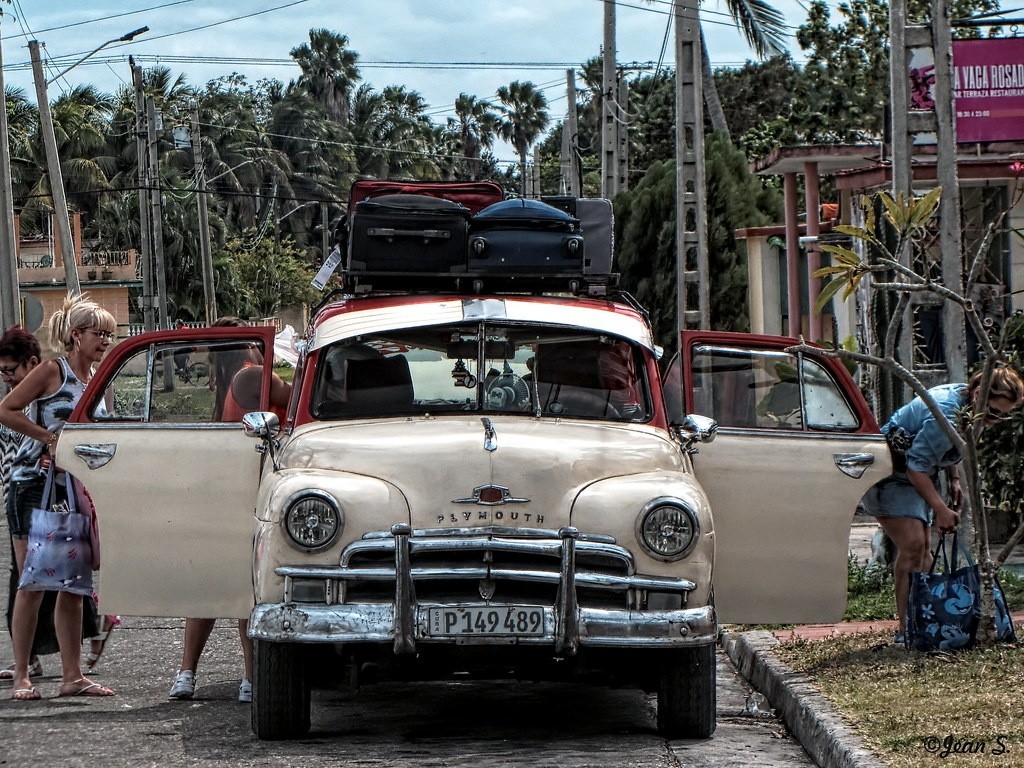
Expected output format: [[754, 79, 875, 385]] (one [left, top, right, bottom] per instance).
[[60, 676, 115, 695], [13, 685, 41, 700]]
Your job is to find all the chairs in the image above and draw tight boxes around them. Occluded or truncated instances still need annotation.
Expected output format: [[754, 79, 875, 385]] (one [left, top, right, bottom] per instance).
[[35, 255, 50, 268], [317, 354, 414, 418]]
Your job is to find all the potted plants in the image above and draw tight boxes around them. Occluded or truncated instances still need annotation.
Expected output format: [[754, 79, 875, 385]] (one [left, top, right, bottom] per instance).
[[88, 268, 97, 280], [102, 261, 113, 279]]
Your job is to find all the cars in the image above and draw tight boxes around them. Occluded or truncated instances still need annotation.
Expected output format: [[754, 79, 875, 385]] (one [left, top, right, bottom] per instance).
[[53, 270, 896, 737]]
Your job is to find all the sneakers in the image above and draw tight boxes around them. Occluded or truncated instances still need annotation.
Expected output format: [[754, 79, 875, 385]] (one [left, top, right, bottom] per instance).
[[894, 631, 910, 648], [168, 670, 196, 700], [238, 677, 252, 702]]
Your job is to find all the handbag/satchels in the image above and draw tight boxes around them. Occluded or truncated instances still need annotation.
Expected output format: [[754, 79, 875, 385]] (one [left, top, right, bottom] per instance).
[[886, 426, 916, 474], [905, 529, 1018, 651], [17, 459, 94, 596]]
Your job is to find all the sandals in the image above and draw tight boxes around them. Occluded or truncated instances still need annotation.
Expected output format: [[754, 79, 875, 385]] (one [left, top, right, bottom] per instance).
[[86, 615, 120, 668], [0, 657, 43, 679]]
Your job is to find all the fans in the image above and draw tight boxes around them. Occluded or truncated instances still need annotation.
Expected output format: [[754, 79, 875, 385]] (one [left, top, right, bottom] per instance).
[[486, 373, 530, 413]]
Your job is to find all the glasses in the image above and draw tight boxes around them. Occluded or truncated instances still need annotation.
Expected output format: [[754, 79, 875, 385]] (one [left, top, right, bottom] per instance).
[[0, 359, 24, 376], [987, 406, 1013, 422], [83, 330, 115, 342]]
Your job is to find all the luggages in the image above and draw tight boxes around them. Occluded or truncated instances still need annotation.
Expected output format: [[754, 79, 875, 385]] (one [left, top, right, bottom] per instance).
[[349, 179, 505, 217], [345, 194, 470, 292], [539, 195, 621, 292], [468, 199, 586, 294]]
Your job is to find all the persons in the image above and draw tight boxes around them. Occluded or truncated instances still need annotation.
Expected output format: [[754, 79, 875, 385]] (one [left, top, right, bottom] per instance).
[[863, 369, 1024, 646], [170, 317, 292, 702], [0, 326, 121, 681], [174, 319, 193, 384], [0, 289, 117, 700]]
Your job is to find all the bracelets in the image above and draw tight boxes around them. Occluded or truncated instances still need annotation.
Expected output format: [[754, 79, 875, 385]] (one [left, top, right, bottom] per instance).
[[46, 435, 56, 449], [951, 476, 960, 480]]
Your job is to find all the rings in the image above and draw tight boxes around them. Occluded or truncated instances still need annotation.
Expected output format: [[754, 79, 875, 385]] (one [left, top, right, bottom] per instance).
[[946, 532, 950, 534]]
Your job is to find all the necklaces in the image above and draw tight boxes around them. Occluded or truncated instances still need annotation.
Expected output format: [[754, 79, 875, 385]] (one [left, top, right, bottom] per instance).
[[73, 368, 89, 392]]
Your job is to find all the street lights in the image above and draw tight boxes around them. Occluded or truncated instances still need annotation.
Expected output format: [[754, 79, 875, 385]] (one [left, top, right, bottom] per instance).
[[27, 25, 154, 298]]
